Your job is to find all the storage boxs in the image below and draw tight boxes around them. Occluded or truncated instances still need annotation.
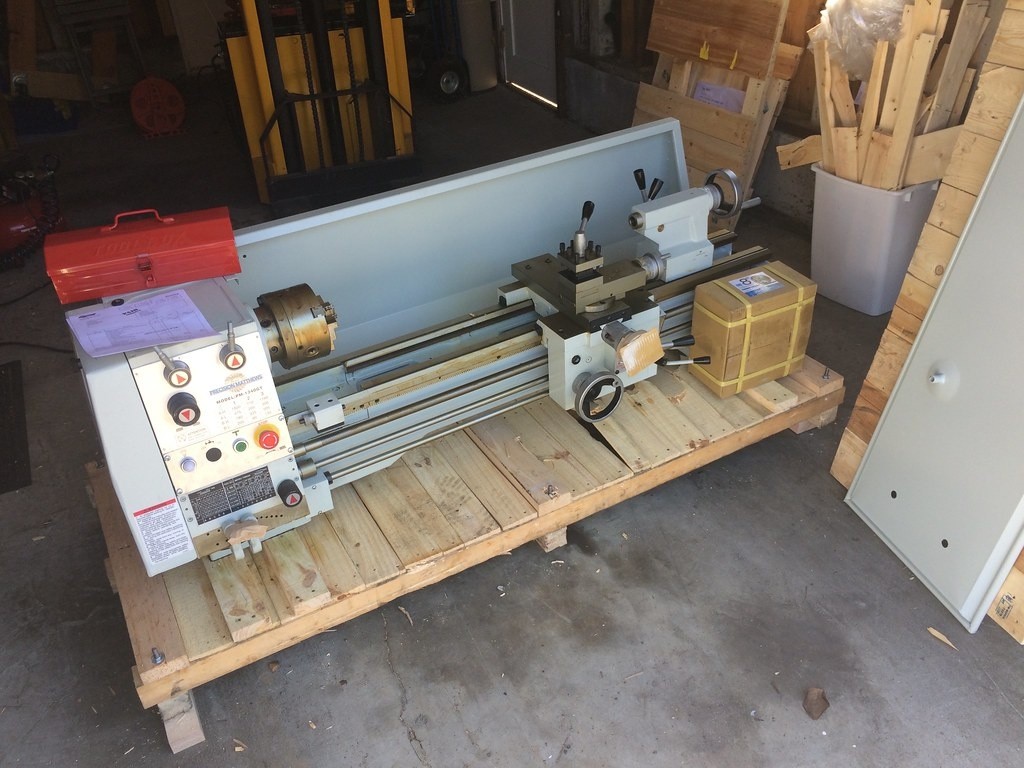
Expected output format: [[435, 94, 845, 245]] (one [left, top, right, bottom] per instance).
[[810, 162, 943, 317], [687, 261, 817, 398]]
